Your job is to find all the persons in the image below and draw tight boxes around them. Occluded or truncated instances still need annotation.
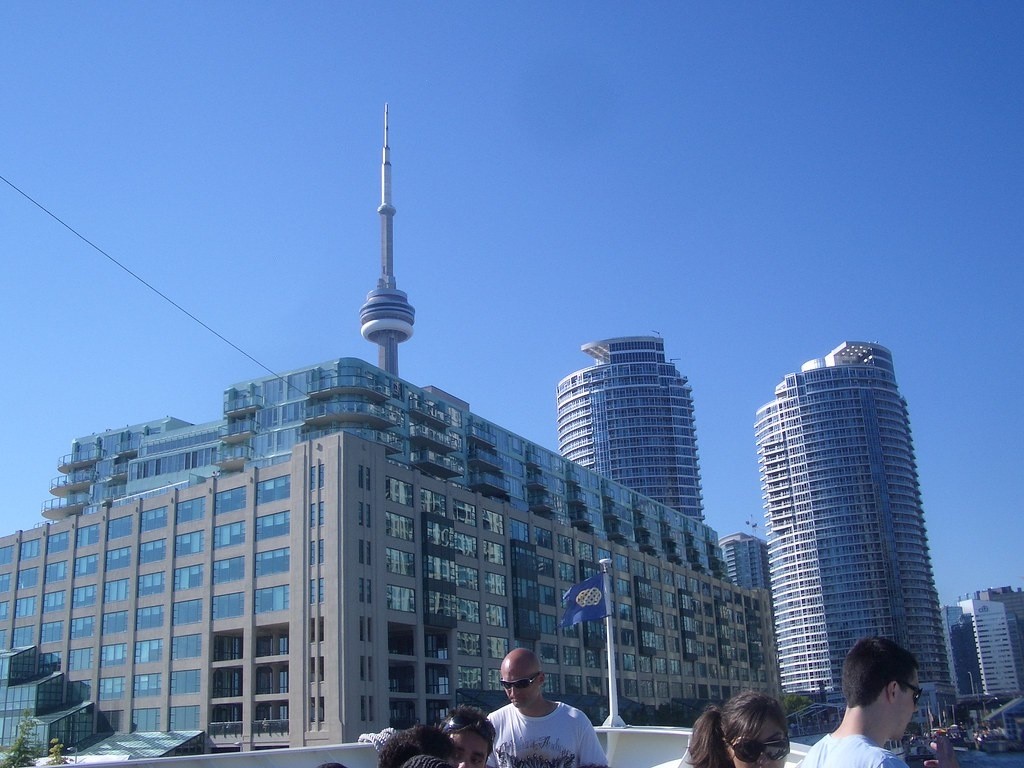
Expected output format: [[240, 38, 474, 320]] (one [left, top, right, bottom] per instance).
[[315, 690, 789, 768], [797, 636, 959, 768], [483, 648, 607, 768]]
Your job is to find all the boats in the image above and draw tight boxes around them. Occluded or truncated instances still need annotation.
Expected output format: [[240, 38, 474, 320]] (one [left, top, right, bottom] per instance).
[[906, 705, 1024, 767]]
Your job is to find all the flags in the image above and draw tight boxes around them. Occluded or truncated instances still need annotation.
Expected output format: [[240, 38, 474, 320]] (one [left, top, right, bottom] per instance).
[[929, 709, 934, 721], [555, 572, 605, 627]]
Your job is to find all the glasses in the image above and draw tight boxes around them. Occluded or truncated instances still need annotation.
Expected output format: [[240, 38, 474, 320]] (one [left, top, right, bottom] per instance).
[[499, 674, 539, 689], [900, 680, 923, 704], [725, 735, 790, 763], [441, 715, 494, 742]]
